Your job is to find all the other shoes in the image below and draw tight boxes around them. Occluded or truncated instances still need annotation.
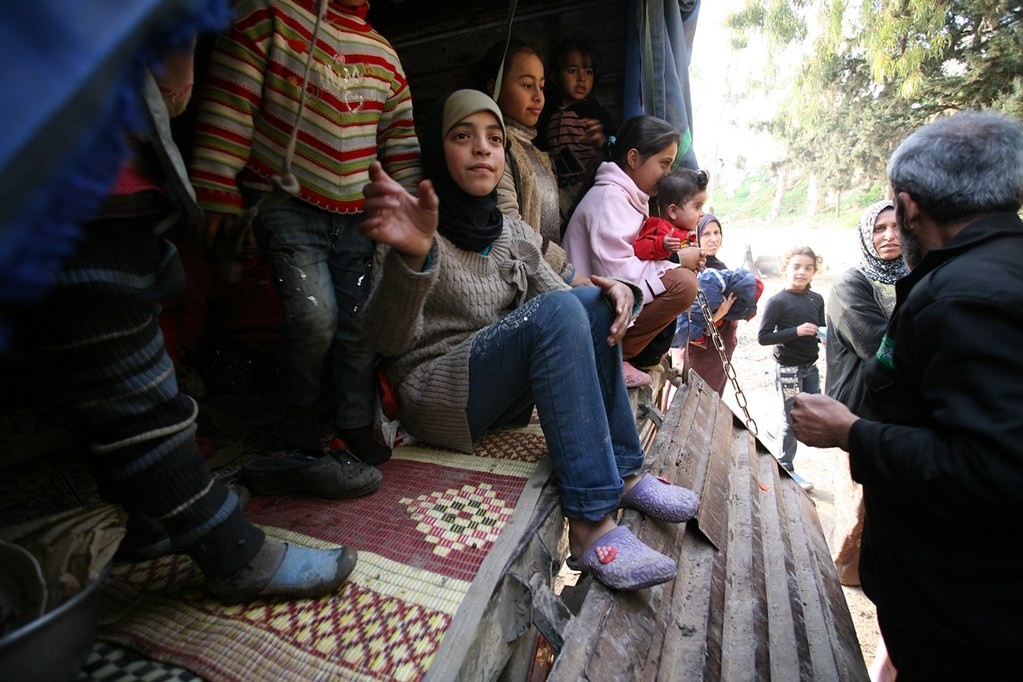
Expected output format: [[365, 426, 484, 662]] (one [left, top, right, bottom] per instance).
[[242, 440, 385, 500], [205, 540, 358, 604], [622, 360, 654, 388]]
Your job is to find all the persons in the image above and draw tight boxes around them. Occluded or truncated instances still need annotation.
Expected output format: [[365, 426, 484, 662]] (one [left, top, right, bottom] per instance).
[[790, 113, 1023, 682], [688, 213, 738, 397], [533, 38, 617, 226], [634, 167, 709, 373], [758, 245, 826, 491], [44, 30, 356, 600], [357, 86, 701, 593], [561, 115, 707, 387], [826, 199, 911, 586], [187, 0, 421, 495], [479, 38, 560, 253]]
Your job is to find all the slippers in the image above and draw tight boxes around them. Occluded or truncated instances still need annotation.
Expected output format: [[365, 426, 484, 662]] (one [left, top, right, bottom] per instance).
[[565, 524, 678, 592], [617, 472, 700, 522]]
